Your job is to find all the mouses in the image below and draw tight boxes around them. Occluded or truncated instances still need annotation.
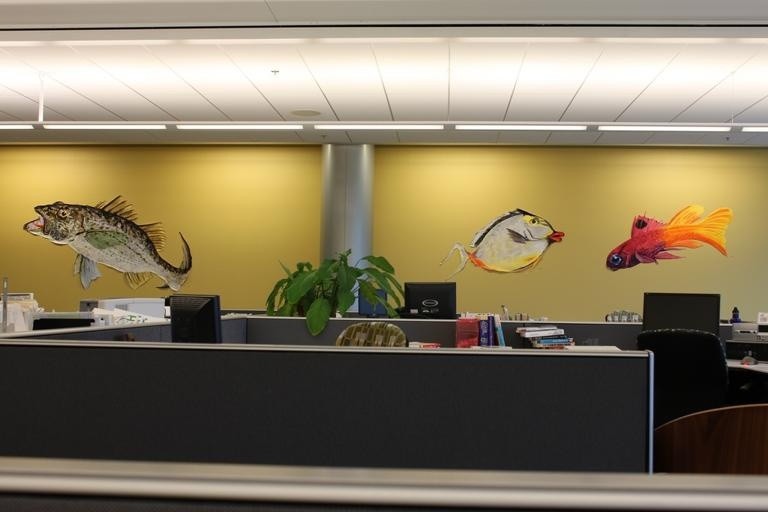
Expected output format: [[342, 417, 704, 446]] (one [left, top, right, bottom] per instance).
[[742, 356, 758, 365]]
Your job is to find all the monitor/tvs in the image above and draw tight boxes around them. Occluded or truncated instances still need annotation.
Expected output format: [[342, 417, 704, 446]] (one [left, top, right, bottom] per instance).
[[642, 293, 720, 335], [170, 294, 222, 343], [404, 282, 456, 319], [33, 318, 95, 330]]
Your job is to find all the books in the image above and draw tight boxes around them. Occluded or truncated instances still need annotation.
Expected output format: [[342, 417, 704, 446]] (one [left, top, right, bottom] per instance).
[[456, 310, 576, 351], [0, 292, 38, 333], [407, 341, 441, 350], [604, 309, 641, 323], [757, 312, 768, 337]]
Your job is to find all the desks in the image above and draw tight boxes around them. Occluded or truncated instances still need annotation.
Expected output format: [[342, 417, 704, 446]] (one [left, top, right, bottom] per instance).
[[726, 358, 768, 376]]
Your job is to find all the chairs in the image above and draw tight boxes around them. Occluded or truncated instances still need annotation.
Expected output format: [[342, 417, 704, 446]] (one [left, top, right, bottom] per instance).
[[655, 402, 768, 475], [637, 329, 730, 429], [336, 322, 407, 347]]
